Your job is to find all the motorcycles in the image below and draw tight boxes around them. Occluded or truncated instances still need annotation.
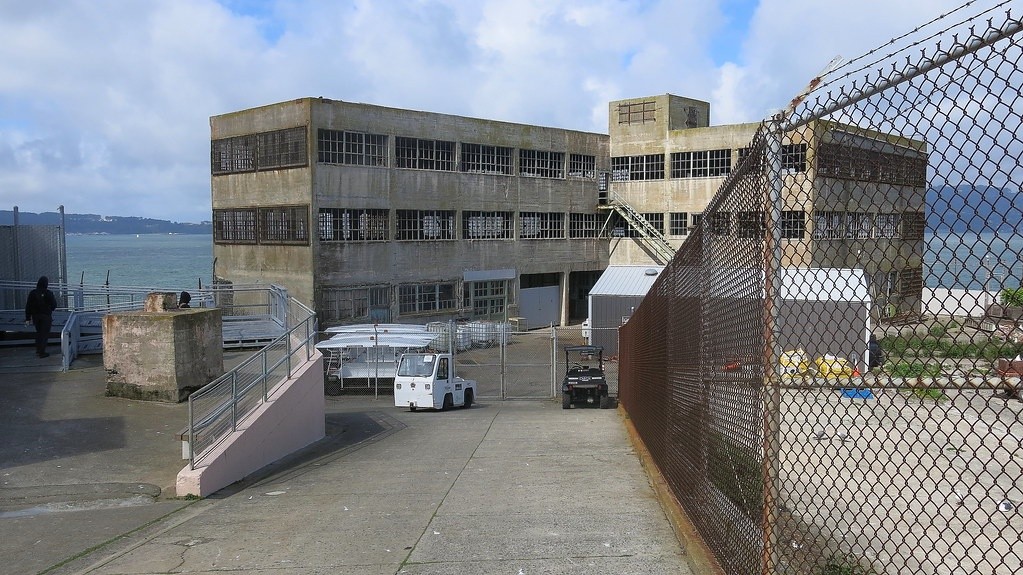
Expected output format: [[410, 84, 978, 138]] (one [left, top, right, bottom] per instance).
[[393, 352, 477, 412]]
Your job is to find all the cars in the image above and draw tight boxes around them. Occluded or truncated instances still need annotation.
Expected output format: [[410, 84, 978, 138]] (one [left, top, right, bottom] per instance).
[[561, 345, 609, 409]]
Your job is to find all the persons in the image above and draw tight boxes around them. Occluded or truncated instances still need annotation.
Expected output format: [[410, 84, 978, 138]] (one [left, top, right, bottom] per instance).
[[25, 276, 57, 359], [179, 291, 191, 308]]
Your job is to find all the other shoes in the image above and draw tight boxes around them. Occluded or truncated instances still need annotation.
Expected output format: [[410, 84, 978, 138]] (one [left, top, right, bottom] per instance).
[[39, 353, 50, 358], [36, 351, 39, 355]]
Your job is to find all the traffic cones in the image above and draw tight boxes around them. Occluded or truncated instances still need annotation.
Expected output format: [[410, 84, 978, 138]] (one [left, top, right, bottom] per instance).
[[853, 358, 861, 377]]
